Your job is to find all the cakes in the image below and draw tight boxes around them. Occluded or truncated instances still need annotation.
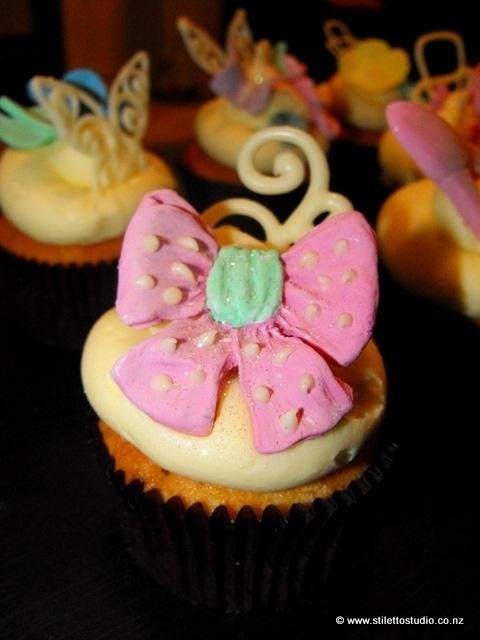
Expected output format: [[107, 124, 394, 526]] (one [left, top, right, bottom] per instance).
[[175, 9, 342, 177], [317, 21, 480, 320], [0, 50, 179, 248], [79, 125, 389, 493]]
